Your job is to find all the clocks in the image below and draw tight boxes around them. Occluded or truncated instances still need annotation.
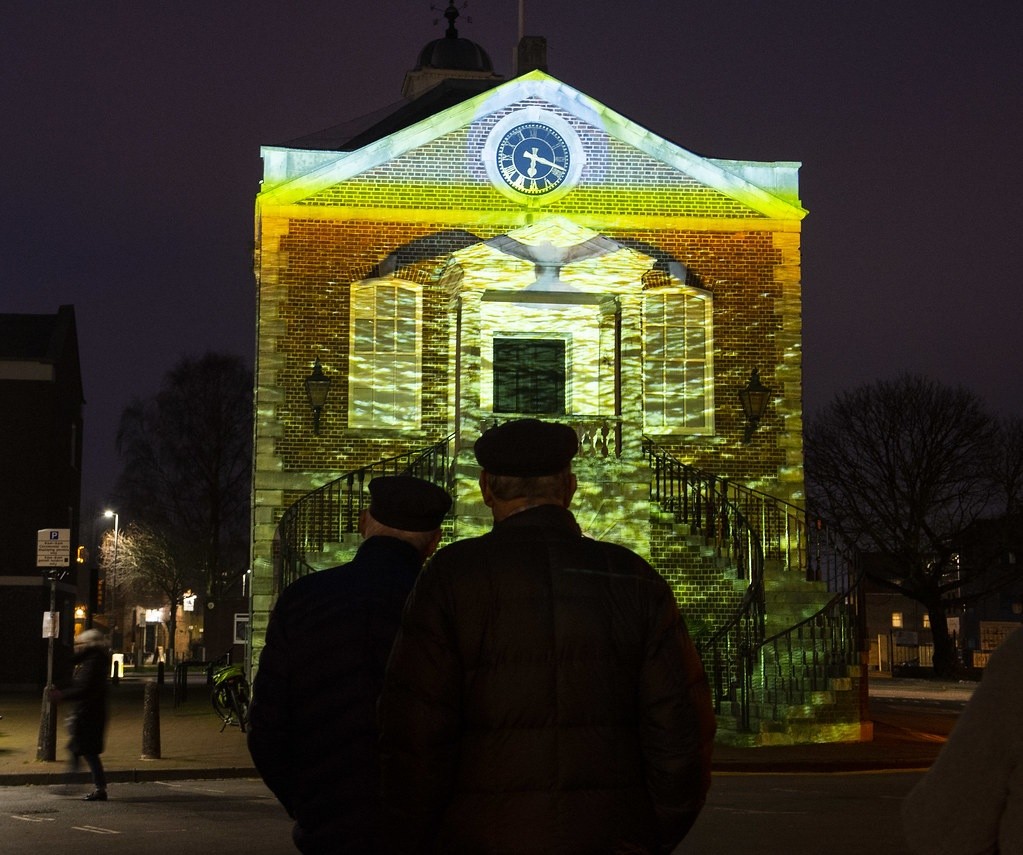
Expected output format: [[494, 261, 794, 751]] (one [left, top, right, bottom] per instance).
[[480, 104, 592, 211]]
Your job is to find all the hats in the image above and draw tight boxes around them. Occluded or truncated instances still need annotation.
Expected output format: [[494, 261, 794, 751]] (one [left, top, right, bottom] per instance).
[[368, 478, 454, 533], [475, 418, 580, 477]]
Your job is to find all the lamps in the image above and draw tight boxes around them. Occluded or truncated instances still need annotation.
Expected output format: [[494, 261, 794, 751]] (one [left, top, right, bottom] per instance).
[[737, 364, 774, 443], [302, 353, 335, 437]]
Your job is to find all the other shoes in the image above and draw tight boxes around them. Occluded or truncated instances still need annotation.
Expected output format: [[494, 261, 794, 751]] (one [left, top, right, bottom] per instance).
[[83, 790, 109, 803]]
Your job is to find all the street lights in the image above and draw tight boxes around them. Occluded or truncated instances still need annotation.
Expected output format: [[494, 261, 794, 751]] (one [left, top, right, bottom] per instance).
[[103, 510, 120, 630]]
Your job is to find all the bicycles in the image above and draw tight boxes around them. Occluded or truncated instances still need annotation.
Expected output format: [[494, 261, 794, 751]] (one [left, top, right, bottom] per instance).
[[210, 667, 249, 734]]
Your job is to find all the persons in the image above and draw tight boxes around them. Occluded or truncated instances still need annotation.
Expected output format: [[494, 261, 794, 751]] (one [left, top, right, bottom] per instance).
[[47, 629, 111, 801], [247, 418, 716, 855]]
